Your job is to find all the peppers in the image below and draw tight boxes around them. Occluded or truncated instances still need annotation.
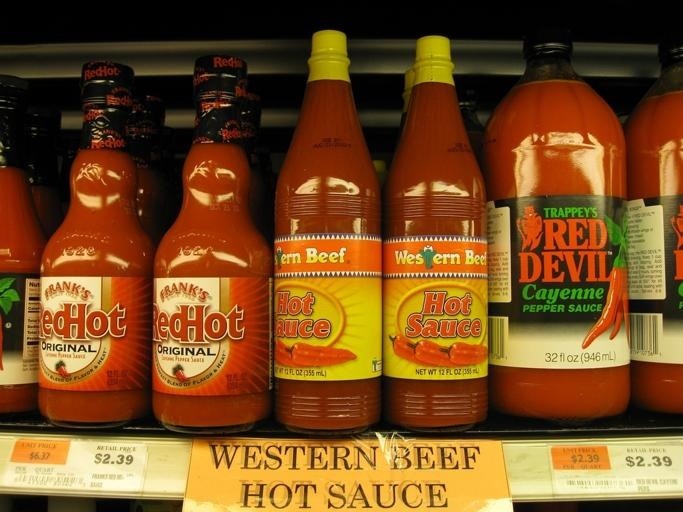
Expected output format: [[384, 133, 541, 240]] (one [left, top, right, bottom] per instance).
[[286, 343, 356, 366], [274, 340, 294, 366], [389, 335, 416, 362], [407, 341, 450, 367], [582, 228, 631, 347], [439, 342, 489, 365]]
